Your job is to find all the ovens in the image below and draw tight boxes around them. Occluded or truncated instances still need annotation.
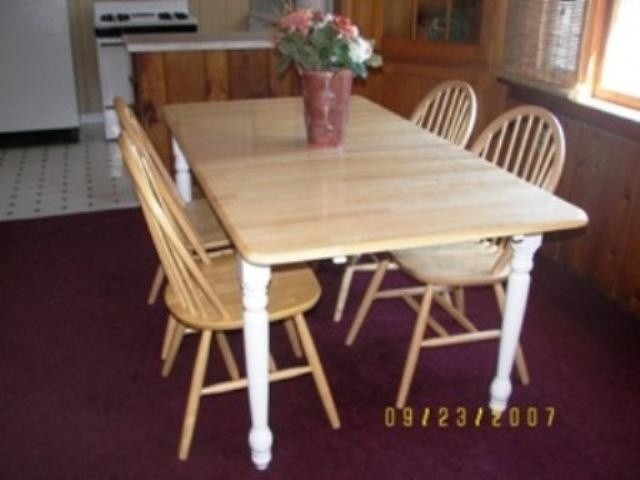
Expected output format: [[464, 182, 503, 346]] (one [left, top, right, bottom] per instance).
[[95, 24, 199, 108]]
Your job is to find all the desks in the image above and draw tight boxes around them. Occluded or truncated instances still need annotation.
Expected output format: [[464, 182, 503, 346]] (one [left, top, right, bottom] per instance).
[[159, 91, 586, 474]]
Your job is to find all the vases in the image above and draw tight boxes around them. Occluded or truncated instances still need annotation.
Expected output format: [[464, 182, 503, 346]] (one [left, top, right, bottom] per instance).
[[302, 68, 355, 149]]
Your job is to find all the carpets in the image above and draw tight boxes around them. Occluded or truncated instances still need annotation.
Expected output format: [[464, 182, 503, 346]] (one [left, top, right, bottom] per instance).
[[2, 208, 640, 480]]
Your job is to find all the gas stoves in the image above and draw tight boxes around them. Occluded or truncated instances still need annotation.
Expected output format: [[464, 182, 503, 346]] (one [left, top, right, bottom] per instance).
[[95, 9, 197, 28]]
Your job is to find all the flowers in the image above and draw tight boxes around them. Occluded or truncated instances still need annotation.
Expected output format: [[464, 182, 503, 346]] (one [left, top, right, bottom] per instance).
[[274, 8, 385, 81]]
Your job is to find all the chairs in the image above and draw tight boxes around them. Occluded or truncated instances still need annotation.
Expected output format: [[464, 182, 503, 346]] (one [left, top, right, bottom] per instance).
[[332, 82, 479, 323], [112, 94, 232, 357], [340, 106, 569, 409], [113, 131, 337, 460]]
